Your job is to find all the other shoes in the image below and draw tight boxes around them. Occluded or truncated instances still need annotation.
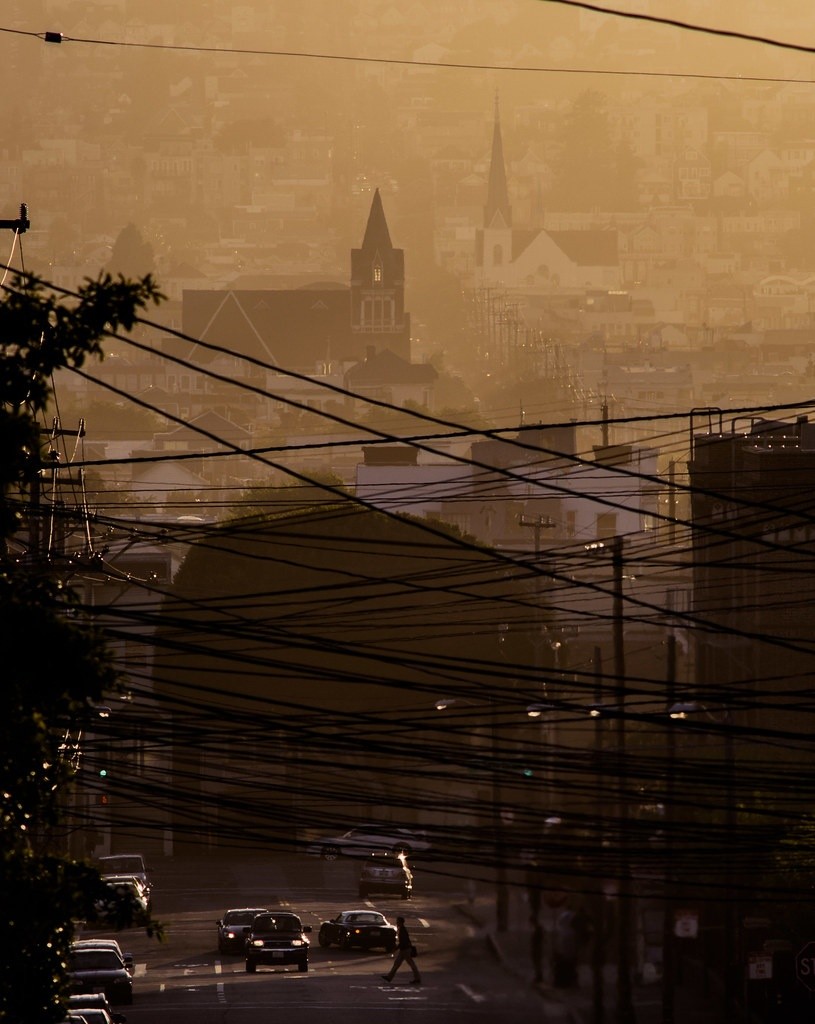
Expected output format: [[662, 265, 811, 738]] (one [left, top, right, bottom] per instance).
[[410, 980, 420, 984], [381, 976, 391, 982]]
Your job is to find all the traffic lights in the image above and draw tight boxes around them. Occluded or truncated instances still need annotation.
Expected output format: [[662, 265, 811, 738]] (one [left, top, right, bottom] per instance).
[[94, 759, 114, 811], [518, 767, 536, 802]]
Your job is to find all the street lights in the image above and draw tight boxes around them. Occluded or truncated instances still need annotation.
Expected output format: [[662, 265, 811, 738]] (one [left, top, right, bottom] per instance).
[[436, 697, 509, 936]]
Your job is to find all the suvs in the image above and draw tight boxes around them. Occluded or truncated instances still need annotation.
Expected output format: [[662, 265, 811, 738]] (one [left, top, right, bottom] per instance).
[[97, 854, 154, 883]]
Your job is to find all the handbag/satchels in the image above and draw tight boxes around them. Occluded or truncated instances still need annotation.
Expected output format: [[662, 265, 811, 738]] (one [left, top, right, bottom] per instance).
[[410, 946, 417, 957]]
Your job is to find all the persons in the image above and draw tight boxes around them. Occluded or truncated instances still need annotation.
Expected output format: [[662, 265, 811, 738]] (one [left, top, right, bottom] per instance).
[[525, 878, 598, 992], [381, 916, 423, 985]]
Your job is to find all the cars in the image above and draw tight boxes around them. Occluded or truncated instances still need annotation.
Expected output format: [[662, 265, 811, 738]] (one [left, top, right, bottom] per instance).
[[307, 824, 432, 860], [352, 855, 414, 900], [216, 907, 268, 955], [65, 940, 133, 1024], [318, 910, 397, 951], [97, 877, 152, 939], [242, 912, 313, 971]]
